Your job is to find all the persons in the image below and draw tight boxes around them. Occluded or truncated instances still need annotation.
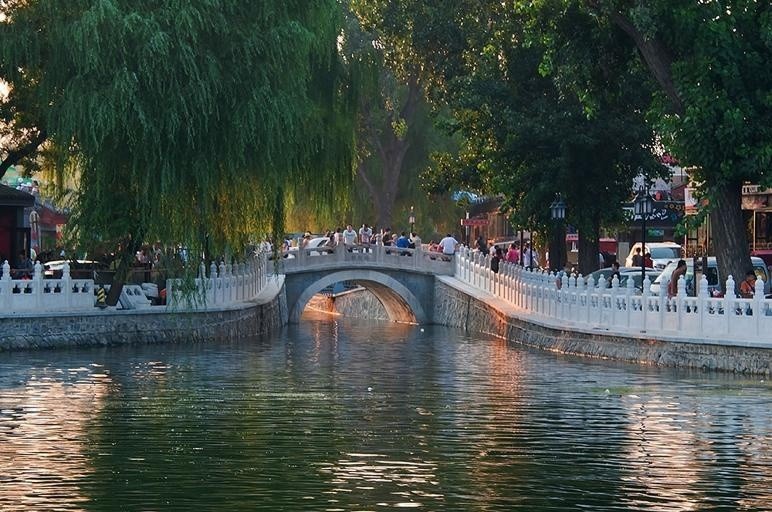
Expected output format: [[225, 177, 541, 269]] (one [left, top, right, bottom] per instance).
[[135, 242, 186, 271], [241, 222, 460, 261], [0, 249, 33, 293], [607, 247, 687, 313], [473, 235, 537, 273], [740, 271, 757, 315], [555, 262, 573, 290]]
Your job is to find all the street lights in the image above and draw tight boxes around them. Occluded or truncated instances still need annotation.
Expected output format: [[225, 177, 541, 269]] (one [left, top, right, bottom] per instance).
[[466, 209, 470, 246], [409, 203, 415, 234], [634, 185, 653, 294], [460, 218, 464, 242], [550, 192, 568, 270]]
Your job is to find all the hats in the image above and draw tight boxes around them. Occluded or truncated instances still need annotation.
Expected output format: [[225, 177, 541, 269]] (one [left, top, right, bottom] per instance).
[[304, 233, 312, 238]]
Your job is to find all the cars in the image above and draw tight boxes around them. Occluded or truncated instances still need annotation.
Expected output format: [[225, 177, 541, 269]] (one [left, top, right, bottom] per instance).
[[584, 241, 771, 299], [288, 237, 359, 259], [29, 259, 106, 279]]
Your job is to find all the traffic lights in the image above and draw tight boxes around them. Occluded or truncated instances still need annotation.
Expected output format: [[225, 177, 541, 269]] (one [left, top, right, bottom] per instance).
[[652, 191, 669, 201]]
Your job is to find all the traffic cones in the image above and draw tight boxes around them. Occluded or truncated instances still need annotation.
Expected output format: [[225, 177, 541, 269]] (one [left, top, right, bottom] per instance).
[[95, 283, 108, 308]]
